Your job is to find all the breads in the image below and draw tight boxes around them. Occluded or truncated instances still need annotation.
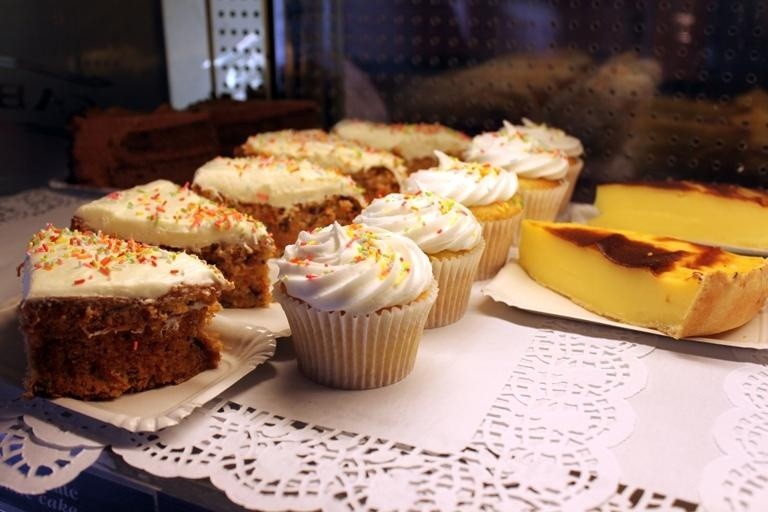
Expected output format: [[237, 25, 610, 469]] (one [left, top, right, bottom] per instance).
[[189, 100, 323, 157], [191, 156, 368, 248], [16, 222, 236, 398], [70, 179, 276, 309], [75, 108, 212, 190], [334, 119, 470, 176], [234, 129, 409, 204]]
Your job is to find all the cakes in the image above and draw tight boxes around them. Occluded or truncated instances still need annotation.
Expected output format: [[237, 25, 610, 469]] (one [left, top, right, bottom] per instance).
[[593, 177, 768, 248], [520, 116, 585, 217], [468, 120, 570, 246], [353, 188, 485, 330], [519, 220, 768, 339], [271, 220, 439, 388], [405, 149, 526, 282]]
